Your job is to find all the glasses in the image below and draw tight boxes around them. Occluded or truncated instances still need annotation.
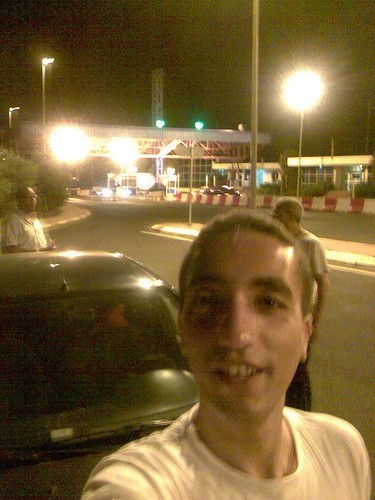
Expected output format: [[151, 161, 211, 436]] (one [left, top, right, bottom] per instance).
[[270, 211, 283, 218], [22, 194, 38, 199]]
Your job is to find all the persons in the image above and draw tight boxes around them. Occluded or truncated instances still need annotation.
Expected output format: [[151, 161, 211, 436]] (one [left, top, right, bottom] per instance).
[[79, 206, 372, 500], [2, 186, 57, 254], [269, 197, 331, 414]]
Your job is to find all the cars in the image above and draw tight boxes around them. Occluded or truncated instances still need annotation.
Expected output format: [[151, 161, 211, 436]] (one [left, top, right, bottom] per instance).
[[0, 250, 200, 499], [202, 185, 240, 196], [114, 175, 179, 195]]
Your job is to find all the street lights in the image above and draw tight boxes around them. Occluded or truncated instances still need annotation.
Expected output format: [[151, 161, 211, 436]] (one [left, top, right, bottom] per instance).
[[8, 107, 20, 128], [41, 58, 54, 130], [281, 73, 319, 197]]
